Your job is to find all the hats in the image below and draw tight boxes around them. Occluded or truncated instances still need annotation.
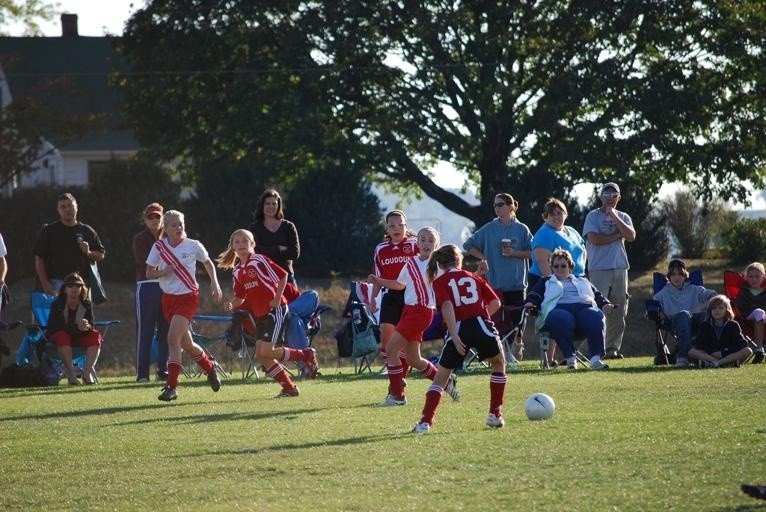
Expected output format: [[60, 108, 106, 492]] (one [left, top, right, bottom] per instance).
[[143, 202, 164, 216], [667, 259, 686, 270], [601, 182, 620, 193], [64, 273, 85, 285]]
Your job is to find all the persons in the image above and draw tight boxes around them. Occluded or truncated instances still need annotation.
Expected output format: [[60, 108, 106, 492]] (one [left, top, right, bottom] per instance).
[[367, 226, 459, 405], [463, 193, 533, 357], [734, 262, 765, 358], [689, 294, 751, 365], [247, 189, 300, 290], [44, 273, 101, 386], [410, 245, 506, 431], [214, 229, 317, 398], [520, 249, 613, 371], [580, 181, 635, 359], [525, 198, 586, 368], [131, 202, 167, 384], [369, 211, 420, 401], [0, 232, 8, 315], [282, 280, 318, 379], [143, 209, 222, 401], [645, 260, 718, 366], [31, 192, 105, 321]]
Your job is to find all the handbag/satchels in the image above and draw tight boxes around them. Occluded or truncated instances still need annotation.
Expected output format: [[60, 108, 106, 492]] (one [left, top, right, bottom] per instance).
[[351, 305, 379, 360], [78, 258, 108, 305]]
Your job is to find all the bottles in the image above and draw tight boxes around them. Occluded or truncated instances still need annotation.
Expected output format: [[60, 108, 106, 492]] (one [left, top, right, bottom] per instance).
[[352, 300, 362, 325]]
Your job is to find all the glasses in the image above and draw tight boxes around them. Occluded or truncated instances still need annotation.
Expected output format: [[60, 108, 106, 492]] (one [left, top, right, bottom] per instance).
[[552, 264, 567, 268], [146, 216, 162, 220], [386, 223, 407, 229], [602, 193, 618, 198], [493, 202, 506, 208], [66, 284, 83, 288]]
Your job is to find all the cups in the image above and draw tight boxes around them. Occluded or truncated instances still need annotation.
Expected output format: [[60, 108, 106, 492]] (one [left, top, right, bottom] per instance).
[[502, 238, 513, 256]]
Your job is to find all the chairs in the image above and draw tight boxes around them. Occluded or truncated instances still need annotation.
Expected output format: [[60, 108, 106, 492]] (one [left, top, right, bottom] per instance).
[[147, 306, 229, 380], [431, 302, 519, 373], [645, 270, 711, 368], [236, 289, 320, 381], [27, 291, 116, 384], [525, 269, 600, 370], [344, 278, 393, 374], [722, 266, 766, 339]]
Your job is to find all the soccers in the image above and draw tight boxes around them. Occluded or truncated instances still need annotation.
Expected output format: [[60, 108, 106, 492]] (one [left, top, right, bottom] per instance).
[[526, 393, 554, 419]]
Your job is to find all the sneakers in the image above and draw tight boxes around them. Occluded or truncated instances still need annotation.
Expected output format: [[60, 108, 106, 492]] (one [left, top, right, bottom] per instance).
[[411, 423, 430, 434], [514, 342, 525, 360], [697, 359, 717, 369], [139, 368, 169, 382], [566, 358, 577, 369], [274, 384, 299, 397], [204, 360, 221, 393], [486, 413, 505, 428], [302, 347, 318, 379], [158, 385, 178, 401], [445, 374, 459, 402], [752, 350, 763, 364], [376, 378, 407, 406], [589, 360, 609, 370], [68, 375, 96, 385], [676, 358, 688, 367], [606, 352, 623, 360]]
